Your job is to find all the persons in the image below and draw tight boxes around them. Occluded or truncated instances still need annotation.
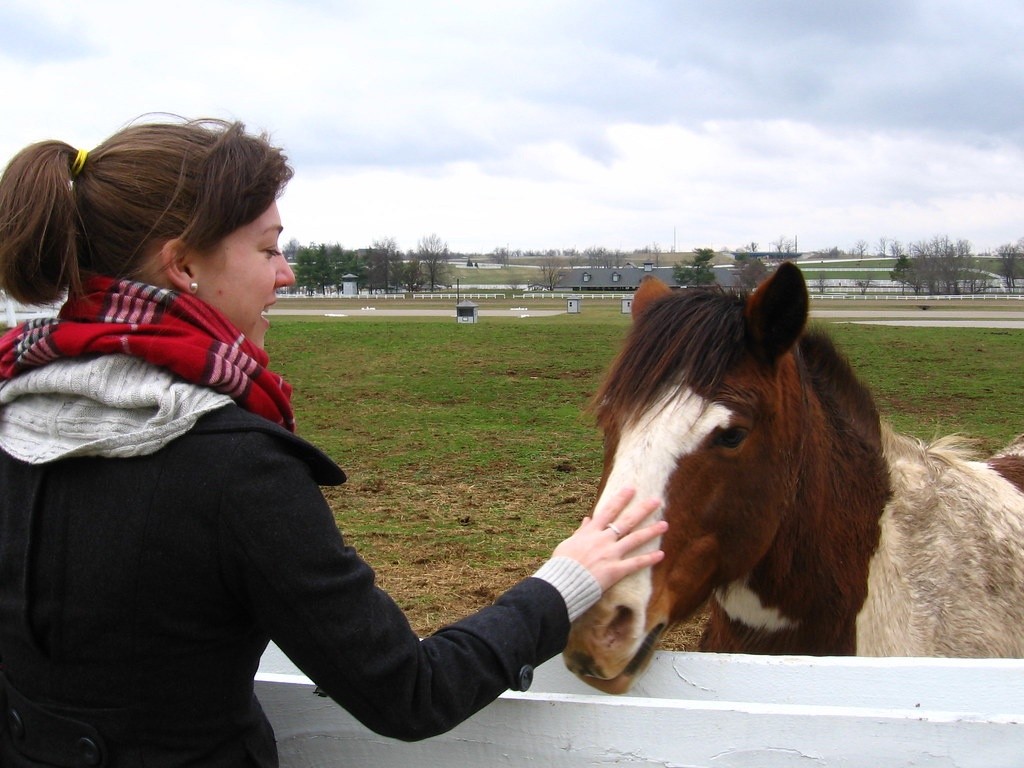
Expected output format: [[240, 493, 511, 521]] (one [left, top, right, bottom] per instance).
[[0, 119, 667, 767]]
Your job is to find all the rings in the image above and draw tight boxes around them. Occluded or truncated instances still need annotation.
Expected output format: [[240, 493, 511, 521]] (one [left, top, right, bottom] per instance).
[[606, 522, 620, 536]]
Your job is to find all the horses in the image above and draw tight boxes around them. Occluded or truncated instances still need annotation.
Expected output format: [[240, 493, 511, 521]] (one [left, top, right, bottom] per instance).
[[558, 260, 1024, 697]]
